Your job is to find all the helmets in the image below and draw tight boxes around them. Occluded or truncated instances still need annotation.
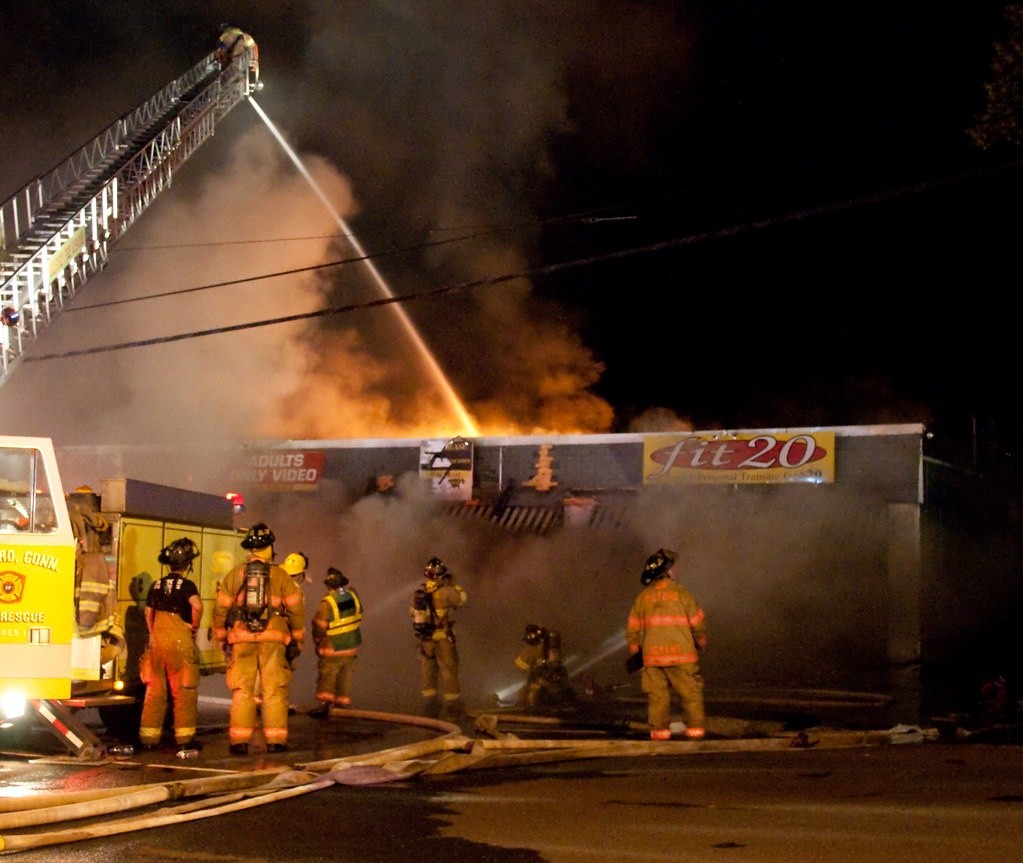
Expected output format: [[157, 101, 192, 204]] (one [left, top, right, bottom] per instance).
[[640, 547, 678, 585], [423, 554, 448, 578], [279, 552, 306, 575], [241, 522, 275, 549], [168, 537, 201, 568], [324, 567, 349, 588]]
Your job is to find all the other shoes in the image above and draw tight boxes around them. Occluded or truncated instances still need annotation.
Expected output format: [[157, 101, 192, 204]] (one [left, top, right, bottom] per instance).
[[229, 743, 250, 757], [308, 700, 332, 718], [266, 743, 289, 753], [178, 739, 203, 752]]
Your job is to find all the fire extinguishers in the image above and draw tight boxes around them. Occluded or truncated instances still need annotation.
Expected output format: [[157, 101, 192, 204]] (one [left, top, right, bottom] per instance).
[[238, 556, 277, 633], [410, 588, 436, 640]]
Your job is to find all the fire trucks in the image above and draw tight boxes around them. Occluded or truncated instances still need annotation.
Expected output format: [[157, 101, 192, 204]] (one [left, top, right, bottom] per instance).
[[0, 434, 267, 748]]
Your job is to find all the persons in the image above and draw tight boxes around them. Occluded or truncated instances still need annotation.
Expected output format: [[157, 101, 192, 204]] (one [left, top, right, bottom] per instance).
[[409, 556, 468, 724], [625, 547, 707, 740], [218, 23, 259, 86], [307, 568, 364, 719], [253, 668, 297, 717], [139, 538, 203, 752], [514, 624, 572, 718], [212, 522, 304, 754]]
